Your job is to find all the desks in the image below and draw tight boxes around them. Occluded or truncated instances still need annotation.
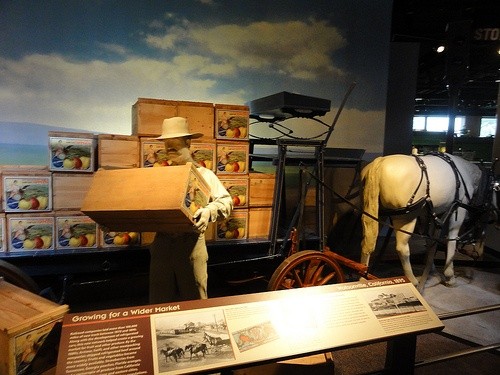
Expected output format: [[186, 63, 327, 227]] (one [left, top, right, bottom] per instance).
[[0, 276, 71, 375]]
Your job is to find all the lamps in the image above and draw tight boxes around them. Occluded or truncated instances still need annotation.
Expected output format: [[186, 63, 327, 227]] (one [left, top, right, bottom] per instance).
[[431, 39, 450, 56]]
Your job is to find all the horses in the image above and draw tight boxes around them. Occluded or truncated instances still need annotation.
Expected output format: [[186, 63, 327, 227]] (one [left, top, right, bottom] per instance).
[[159, 346, 185, 363], [185, 343, 210, 361], [358, 147, 500, 289]]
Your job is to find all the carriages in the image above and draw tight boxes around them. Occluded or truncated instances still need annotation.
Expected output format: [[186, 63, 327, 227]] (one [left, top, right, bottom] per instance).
[[0, 134, 500, 298]]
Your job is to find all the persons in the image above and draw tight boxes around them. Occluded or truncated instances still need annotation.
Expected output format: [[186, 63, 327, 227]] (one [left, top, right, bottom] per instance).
[[101, 116, 233, 305]]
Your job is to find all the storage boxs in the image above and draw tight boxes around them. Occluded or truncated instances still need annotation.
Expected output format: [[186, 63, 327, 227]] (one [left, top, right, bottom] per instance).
[[0, 97, 277, 259]]
